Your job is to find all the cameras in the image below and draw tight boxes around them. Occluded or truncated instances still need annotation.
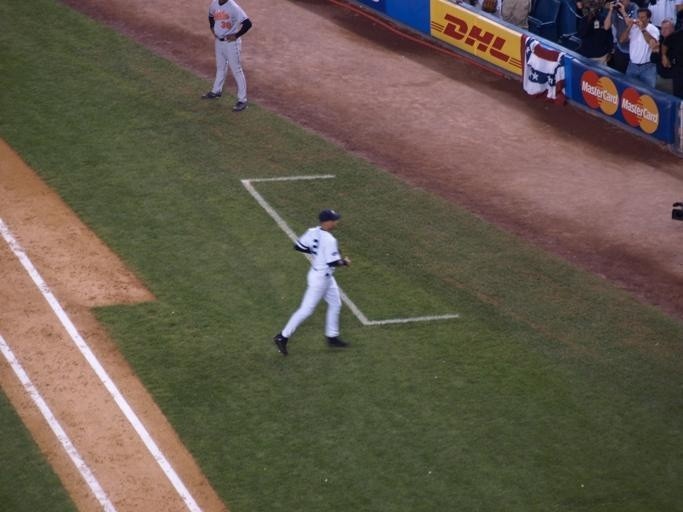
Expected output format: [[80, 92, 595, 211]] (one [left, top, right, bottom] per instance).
[[633, 18, 639, 24], [612, 1, 622, 8]]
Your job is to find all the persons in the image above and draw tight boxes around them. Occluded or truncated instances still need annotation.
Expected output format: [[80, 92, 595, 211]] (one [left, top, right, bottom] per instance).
[[456, 0, 683, 88], [202, 1, 252, 111], [272, 209, 352, 357]]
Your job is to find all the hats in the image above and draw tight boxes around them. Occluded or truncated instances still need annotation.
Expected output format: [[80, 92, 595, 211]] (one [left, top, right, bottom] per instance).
[[319, 209, 340, 222]]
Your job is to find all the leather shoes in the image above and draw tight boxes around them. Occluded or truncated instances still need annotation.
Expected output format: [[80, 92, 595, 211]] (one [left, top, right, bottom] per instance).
[[273, 334, 288, 355], [328, 337, 350, 347], [201, 91, 221, 99], [233, 102, 246, 111]]
